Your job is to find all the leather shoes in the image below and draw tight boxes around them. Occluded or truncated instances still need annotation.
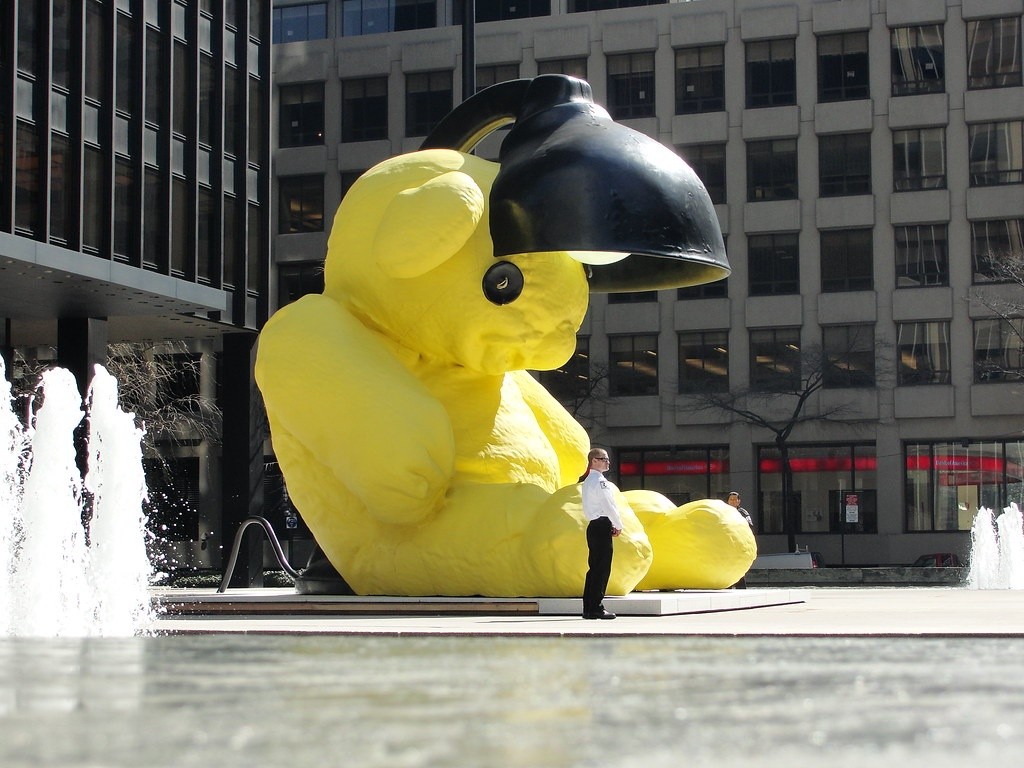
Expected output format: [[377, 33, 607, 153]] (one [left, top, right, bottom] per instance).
[[582, 609, 616, 620]]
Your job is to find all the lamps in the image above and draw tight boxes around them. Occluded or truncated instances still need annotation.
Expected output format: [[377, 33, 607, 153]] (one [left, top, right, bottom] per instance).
[[419, 75, 732, 293]]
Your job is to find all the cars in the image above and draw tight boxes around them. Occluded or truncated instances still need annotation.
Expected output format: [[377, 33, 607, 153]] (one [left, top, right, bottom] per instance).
[[811, 552, 826, 569], [912, 553, 959, 567]]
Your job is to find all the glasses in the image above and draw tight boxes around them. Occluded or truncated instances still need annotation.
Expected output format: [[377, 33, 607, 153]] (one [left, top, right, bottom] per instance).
[[595, 458, 609, 461]]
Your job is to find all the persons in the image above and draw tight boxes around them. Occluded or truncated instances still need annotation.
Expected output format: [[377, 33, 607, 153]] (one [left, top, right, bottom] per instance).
[[724, 492, 753, 589], [582, 447, 623, 620]]
